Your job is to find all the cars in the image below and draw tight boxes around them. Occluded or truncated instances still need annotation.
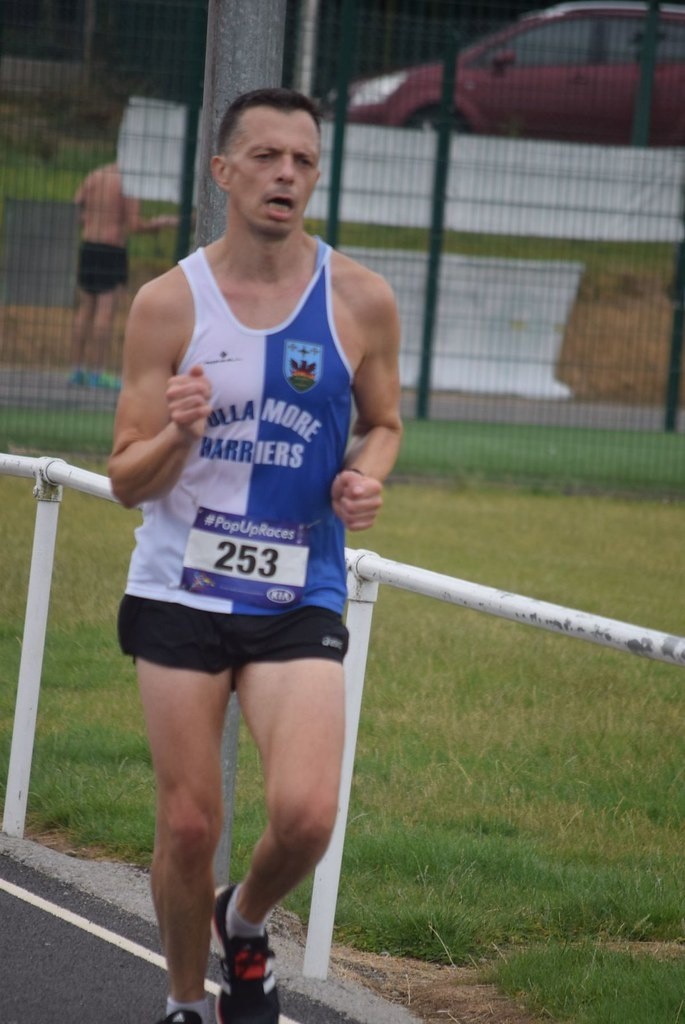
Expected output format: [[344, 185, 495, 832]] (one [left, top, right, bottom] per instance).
[[321, 2, 684, 149]]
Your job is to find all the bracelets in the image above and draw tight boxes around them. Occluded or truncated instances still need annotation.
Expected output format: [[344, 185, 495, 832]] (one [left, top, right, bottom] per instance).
[[346, 469, 361, 473]]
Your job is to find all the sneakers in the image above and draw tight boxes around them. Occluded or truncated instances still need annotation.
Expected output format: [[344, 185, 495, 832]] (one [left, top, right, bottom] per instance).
[[68, 371, 85, 384], [87, 372, 122, 391], [156, 1010, 202, 1024], [211, 884, 280, 1024]]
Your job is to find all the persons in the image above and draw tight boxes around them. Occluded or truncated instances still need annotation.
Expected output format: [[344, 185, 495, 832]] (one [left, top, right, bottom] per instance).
[[108, 87, 402, 1024], [66, 160, 178, 390]]
